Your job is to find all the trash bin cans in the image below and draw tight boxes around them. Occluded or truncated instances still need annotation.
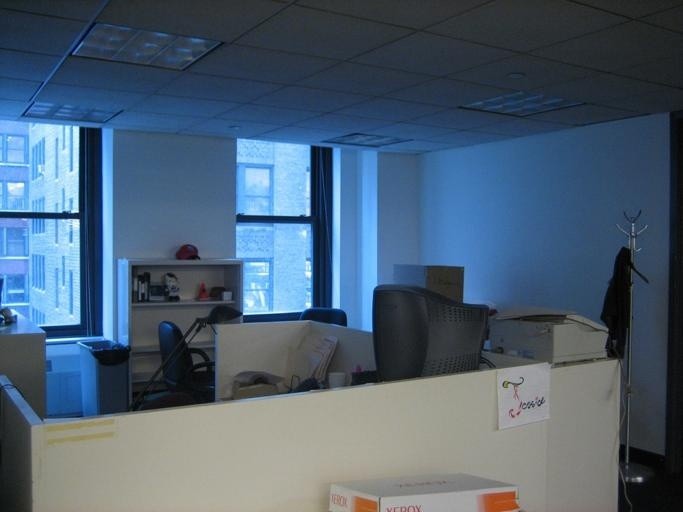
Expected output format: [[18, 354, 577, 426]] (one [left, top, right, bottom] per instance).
[[76, 340, 131, 417]]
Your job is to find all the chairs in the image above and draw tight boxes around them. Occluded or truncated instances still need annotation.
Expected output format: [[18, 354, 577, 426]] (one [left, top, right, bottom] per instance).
[[300, 308, 346, 326], [159, 321, 216, 397]]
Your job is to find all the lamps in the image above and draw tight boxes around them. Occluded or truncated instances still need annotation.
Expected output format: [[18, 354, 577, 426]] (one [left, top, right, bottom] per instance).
[[130, 305, 242, 409]]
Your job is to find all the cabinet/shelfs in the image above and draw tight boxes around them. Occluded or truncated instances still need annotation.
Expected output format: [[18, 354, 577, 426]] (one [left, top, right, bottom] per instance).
[[117, 257, 244, 407]]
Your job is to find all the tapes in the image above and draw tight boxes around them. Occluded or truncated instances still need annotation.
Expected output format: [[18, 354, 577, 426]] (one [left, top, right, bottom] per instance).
[[249, 374, 268, 385]]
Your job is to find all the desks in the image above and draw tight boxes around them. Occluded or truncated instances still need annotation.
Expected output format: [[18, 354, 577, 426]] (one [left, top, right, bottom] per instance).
[[0, 309, 47, 421]]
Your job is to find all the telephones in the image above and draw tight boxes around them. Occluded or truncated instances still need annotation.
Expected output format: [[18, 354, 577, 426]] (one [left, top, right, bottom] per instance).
[[291, 377, 319, 393]]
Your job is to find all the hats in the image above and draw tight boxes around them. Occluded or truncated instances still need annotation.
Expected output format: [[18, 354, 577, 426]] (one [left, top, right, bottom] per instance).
[[175, 244, 201, 261]]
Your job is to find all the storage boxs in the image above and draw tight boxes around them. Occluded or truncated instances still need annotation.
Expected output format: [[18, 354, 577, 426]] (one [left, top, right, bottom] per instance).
[[329, 474, 521, 512], [392, 264, 465, 302]]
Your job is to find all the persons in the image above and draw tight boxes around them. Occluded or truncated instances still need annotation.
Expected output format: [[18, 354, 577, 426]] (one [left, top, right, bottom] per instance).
[[163, 272, 180, 303]]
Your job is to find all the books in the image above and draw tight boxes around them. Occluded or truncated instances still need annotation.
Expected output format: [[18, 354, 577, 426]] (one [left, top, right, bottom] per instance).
[[132, 266, 151, 304]]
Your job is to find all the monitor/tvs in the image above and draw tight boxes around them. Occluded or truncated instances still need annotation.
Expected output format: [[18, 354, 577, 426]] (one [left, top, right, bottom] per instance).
[[371, 283, 488, 381]]
